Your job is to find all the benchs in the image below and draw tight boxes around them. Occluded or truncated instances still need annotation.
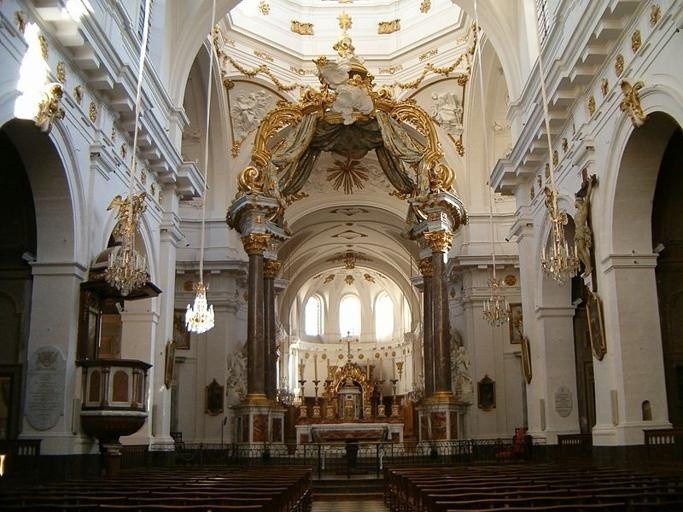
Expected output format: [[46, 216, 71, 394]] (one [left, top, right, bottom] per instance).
[[1, 464, 315, 512], [382, 461, 682, 511]]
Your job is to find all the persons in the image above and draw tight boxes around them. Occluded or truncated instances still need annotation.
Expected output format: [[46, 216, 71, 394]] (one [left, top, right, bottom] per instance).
[[572, 178, 595, 280]]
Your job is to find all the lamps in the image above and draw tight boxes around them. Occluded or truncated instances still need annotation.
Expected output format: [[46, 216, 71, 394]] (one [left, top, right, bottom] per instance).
[[106, 0, 215, 334], [471, 0, 583, 329]]
